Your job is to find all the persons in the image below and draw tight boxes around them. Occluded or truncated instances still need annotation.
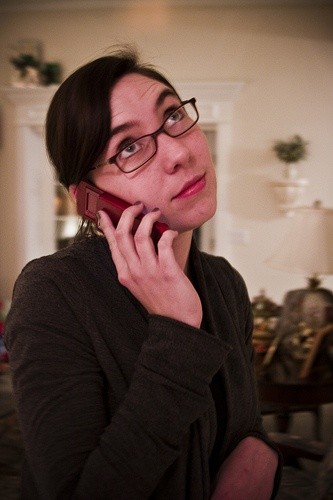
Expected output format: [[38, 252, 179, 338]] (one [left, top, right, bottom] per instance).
[[4, 43, 282, 500]]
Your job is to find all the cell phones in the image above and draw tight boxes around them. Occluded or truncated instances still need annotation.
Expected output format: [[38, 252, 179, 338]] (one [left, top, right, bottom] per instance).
[[75, 179, 169, 256]]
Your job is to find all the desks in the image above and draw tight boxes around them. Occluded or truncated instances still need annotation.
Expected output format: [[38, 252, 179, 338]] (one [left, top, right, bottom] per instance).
[[252, 387, 332, 446]]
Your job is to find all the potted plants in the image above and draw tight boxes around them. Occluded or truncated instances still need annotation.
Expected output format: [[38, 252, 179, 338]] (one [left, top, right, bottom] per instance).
[[270, 134, 308, 216]]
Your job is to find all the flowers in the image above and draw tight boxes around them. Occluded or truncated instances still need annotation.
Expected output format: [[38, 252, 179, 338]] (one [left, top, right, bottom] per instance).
[[11, 50, 62, 87]]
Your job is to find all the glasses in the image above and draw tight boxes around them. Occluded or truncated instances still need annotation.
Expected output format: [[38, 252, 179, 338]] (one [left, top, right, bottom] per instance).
[[89, 97, 199, 174]]
